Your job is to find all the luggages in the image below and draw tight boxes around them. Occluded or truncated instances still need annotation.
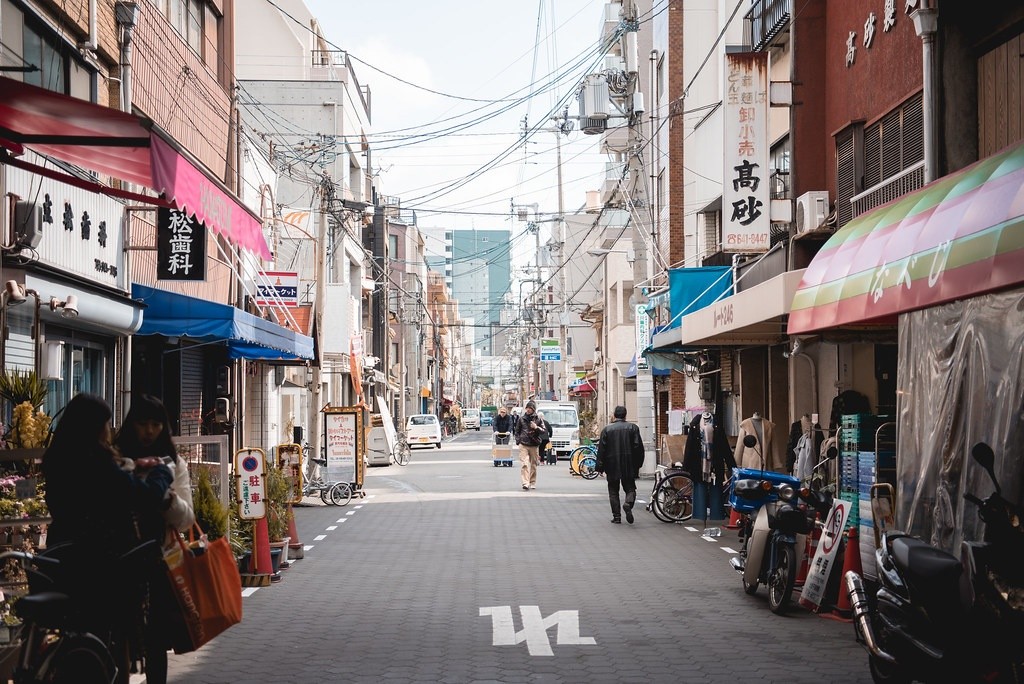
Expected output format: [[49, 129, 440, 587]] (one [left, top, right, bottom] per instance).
[[546, 440, 557, 465]]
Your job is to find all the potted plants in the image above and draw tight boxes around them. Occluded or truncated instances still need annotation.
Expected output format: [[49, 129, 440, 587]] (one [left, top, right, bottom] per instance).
[[188, 459, 304, 579], [0, 497, 52, 546]]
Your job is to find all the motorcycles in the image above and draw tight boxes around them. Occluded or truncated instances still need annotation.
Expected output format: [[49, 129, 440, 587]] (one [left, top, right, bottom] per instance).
[[845, 442, 1024, 684], [729, 435, 838, 615]]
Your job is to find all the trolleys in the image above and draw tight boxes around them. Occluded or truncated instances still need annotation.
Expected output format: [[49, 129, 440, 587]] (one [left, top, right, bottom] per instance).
[[492, 433, 514, 467]]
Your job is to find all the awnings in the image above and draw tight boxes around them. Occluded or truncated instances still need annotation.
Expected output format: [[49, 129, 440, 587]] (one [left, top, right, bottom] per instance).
[[132, 284, 314, 362], [787, 138, 1024, 334], [1, 76, 272, 260], [681, 268, 806, 345], [576, 381, 596, 392], [652, 240, 781, 349]]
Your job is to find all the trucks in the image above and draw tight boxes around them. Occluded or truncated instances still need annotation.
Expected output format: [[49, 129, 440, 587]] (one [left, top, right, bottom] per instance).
[[537, 401, 580, 459], [481, 406, 498, 420]]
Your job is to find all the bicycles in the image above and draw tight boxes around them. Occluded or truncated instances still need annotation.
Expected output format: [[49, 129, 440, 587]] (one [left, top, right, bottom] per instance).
[[0, 552, 119, 683], [568, 438, 600, 480], [393, 429, 411, 466], [646, 466, 733, 522]]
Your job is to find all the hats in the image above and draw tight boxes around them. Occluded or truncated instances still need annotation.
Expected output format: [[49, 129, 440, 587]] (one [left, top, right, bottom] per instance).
[[525, 400, 536, 412]]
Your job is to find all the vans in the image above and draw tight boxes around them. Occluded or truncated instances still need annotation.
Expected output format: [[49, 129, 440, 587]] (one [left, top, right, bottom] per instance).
[[479, 411, 493, 426], [406, 414, 441, 448], [460, 409, 481, 431]]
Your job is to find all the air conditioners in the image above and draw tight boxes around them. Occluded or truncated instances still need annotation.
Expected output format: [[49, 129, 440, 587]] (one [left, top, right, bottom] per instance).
[[795, 190, 830, 235]]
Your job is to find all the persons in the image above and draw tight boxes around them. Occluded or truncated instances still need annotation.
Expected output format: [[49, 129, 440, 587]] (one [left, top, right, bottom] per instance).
[[493, 408, 511, 467], [683, 412, 737, 529], [113, 395, 194, 684], [734, 411, 783, 471], [786, 415, 825, 476], [538, 413, 553, 465], [40, 392, 173, 684], [595, 406, 645, 524], [512, 411, 519, 437], [514, 400, 546, 489], [449, 414, 456, 425]]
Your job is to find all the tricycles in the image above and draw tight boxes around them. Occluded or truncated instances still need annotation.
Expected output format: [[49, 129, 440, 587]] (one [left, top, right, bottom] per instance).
[[301, 445, 354, 506]]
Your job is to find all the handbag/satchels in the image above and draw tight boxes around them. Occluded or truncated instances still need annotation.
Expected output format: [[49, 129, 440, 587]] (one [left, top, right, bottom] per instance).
[[156, 517, 241, 655]]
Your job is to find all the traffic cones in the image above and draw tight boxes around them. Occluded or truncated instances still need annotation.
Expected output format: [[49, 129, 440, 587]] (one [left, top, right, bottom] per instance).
[[820, 527, 864, 623], [720, 506, 742, 530], [284, 505, 299, 543], [249, 516, 281, 582]]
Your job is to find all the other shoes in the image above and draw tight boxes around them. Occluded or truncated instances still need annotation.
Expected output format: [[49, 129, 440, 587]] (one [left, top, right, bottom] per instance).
[[530, 482, 535, 488], [622, 504, 634, 524], [611, 517, 621, 523], [523, 485, 529, 490]]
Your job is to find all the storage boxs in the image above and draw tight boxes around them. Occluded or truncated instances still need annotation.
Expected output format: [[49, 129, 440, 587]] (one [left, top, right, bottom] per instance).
[[728, 467, 801, 513]]
